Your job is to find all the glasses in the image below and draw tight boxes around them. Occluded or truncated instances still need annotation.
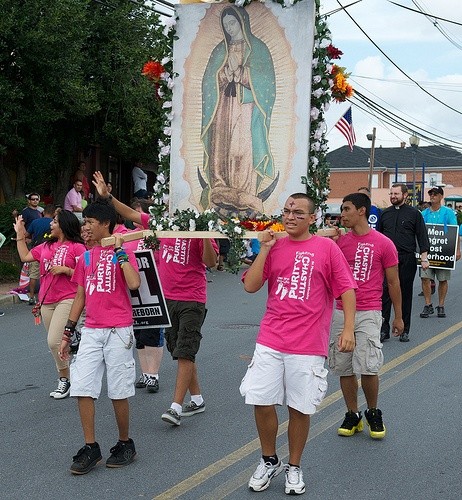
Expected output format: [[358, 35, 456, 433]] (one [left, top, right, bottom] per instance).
[[282, 209, 314, 217], [429, 193, 437, 196], [388, 193, 399, 196], [30, 198, 39, 201]]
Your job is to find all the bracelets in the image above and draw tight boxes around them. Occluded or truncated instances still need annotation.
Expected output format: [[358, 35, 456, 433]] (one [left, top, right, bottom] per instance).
[[62, 319, 77, 343], [114, 247, 129, 264], [17, 238, 24, 241], [69, 269, 71, 275]]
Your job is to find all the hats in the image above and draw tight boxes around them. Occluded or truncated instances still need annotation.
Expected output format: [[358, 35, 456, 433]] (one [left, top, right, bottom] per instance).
[[428, 187, 444, 194]]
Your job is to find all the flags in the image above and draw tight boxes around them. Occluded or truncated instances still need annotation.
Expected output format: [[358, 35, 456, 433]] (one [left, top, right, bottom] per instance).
[[335, 106, 356, 151]]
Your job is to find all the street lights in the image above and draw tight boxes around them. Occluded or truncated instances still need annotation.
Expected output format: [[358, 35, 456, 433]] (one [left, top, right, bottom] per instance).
[[408, 131, 420, 211]]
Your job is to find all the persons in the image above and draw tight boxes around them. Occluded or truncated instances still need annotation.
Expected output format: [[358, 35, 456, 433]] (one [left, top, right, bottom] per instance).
[[73, 161, 91, 202], [133, 160, 151, 199], [113, 211, 127, 235], [326, 193, 405, 439], [414, 186, 462, 317], [12, 193, 56, 306], [357, 187, 383, 231], [237, 193, 358, 493], [58, 200, 141, 474], [63, 180, 85, 212], [323, 213, 350, 235], [12, 210, 86, 399], [111, 199, 167, 392], [375, 184, 429, 341], [91, 171, 220, 426], [217, 238, 231, 271]]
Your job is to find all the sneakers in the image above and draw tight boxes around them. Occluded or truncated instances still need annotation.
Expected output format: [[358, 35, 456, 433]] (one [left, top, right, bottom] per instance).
[[379, 331, 390, 342], [106, 438, 137, 467], [54, 377, 70, 399], [49, 388, 58, 397], [337, 409, 363, 436], [70, 442, 102, 474], [145, 376, 159, 392], [420, 303, 434, 317], [400, 332, 409, 342], [161, 408, 181, 426], [136, 373, 148, 388], [248, 458, 284, 491], [181, 400, 206, 416], [435, 305, 446, 317], [364, 408, 386, 439], [284, 466, 306, 495]]
